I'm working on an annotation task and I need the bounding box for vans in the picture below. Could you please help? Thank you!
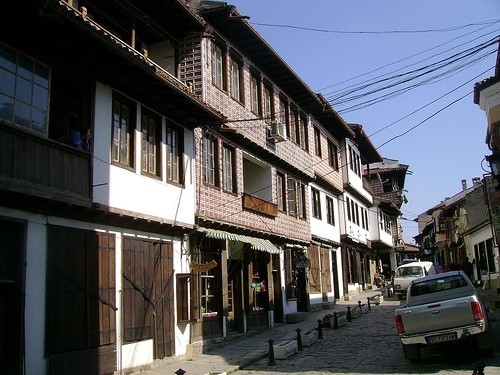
[393,261,437,301]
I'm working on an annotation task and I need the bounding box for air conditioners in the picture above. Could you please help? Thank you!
[270,120,287,142]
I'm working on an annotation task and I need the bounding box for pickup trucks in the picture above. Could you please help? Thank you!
[395,270,495,365]
